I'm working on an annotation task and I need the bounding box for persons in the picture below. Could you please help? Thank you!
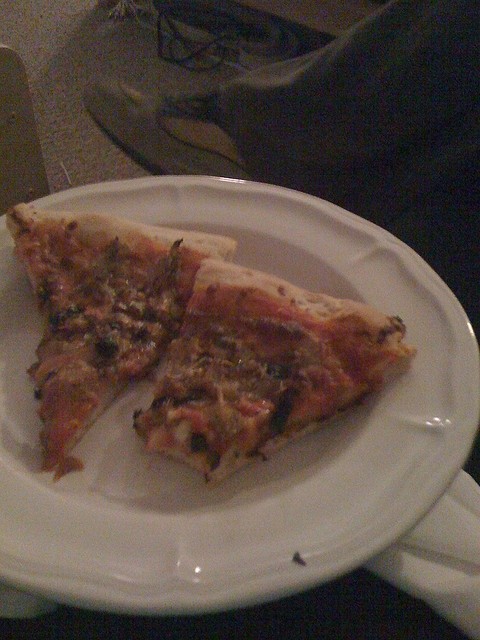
[88,1,478,343]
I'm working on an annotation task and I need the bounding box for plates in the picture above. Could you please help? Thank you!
[0,174,479,617]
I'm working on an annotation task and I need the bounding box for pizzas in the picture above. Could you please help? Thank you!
[5,208,237,473]
[134,259,419,494]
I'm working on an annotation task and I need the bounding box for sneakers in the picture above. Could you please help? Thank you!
[83,79,255,178]
